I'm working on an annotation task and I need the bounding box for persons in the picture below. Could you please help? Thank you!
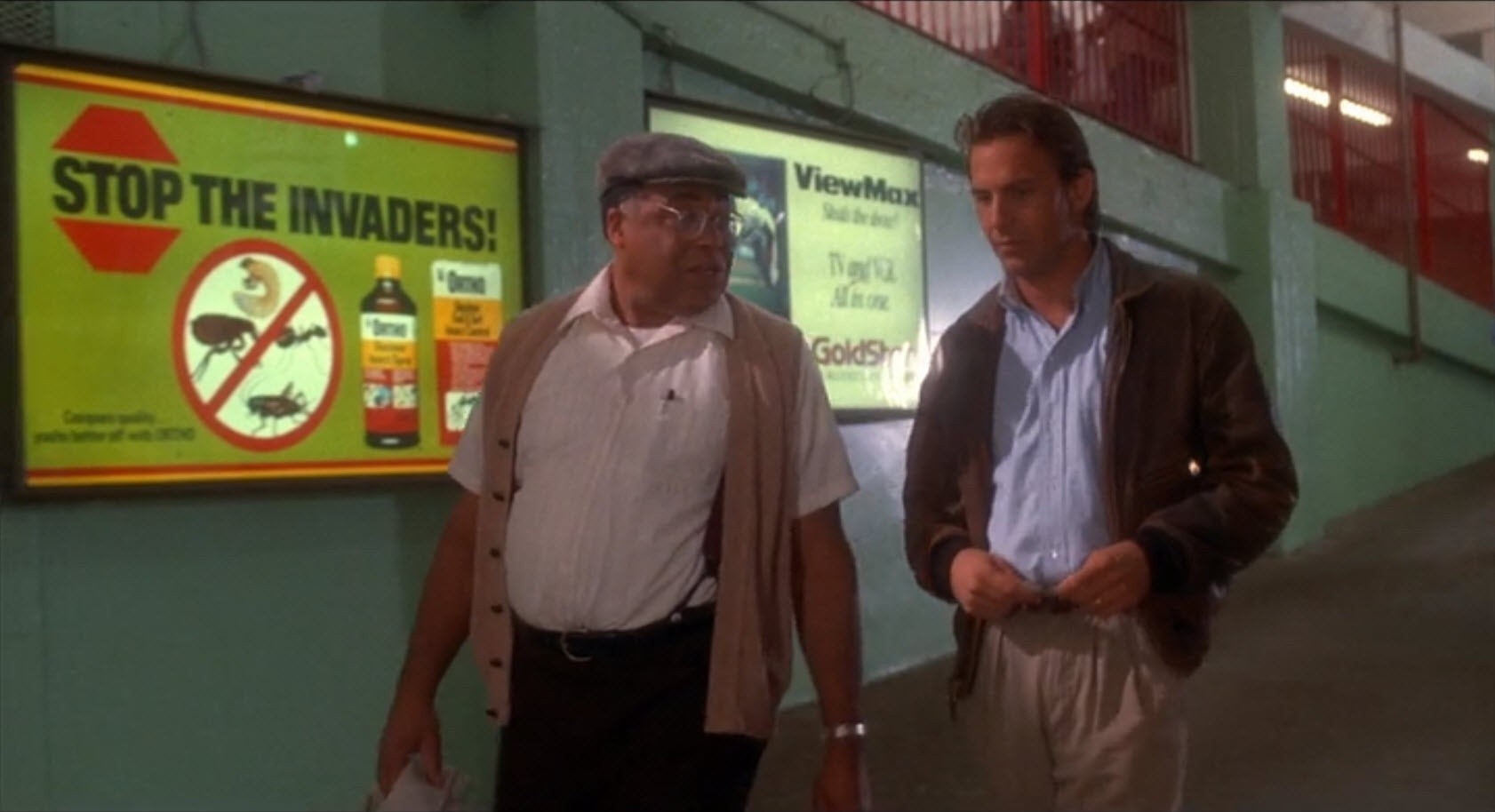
[903,92,1299,812]
[377,132,875,812]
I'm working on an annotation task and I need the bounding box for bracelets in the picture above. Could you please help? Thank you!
[821,721,867,740]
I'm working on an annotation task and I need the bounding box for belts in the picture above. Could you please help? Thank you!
[518,603,718,662]
[1011,593,1077,616]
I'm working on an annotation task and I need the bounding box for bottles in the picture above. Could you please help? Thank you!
[359,254,422,450]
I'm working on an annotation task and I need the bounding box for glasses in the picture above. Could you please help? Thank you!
[615,193,744,246]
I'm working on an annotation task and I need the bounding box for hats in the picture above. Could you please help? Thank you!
[597,134,744,201]
